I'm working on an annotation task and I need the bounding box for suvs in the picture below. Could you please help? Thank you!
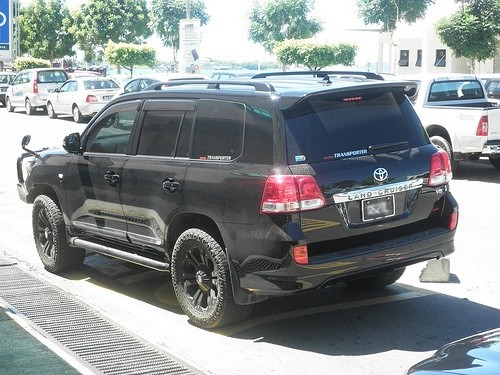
[17,71,460,330]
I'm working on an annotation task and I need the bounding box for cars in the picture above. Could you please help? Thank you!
[480,78,500,100]
[67,73,98,78]
[0,72,17,105]
[109,74,209,128]
[45,76,124,123]
[211,71,257,80]
[326,72,394,81]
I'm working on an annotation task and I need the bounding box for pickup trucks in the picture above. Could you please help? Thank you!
[384,76,500,171]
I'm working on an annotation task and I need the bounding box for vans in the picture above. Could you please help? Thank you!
[5,68,72,115]
